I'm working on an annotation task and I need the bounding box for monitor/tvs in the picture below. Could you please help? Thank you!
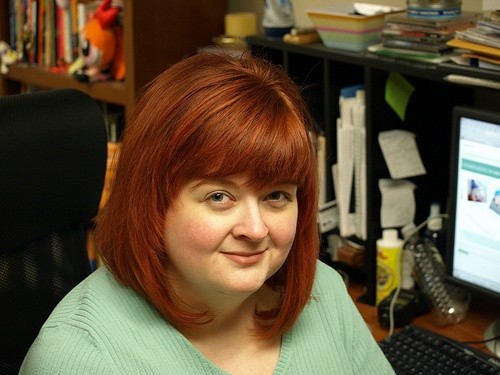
[444,107,500,297]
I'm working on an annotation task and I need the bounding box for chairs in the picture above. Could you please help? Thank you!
[0,88,108,375]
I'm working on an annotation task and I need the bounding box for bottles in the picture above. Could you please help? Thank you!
[376,229,404,308]
[401,222,467,326]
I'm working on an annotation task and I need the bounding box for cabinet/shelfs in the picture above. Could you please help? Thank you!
[0,0,228,129]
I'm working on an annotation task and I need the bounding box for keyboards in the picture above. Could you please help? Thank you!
[378,325,500,375]
